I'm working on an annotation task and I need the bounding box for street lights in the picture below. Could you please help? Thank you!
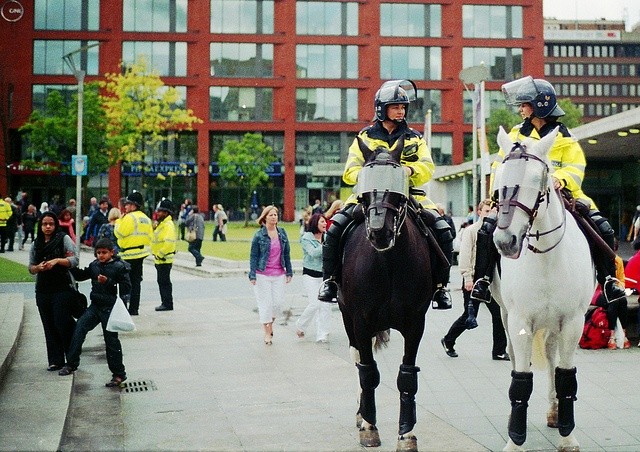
[58,37,106,270]
[460,61,490,222]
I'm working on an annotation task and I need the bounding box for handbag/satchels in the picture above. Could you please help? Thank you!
[184,214,196,242]
[57,286,87,317]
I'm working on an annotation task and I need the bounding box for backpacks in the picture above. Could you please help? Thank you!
[579,308,611,350]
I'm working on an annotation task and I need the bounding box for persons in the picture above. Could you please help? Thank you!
[180,198,194,233]
[184,205,205,267]
[247,204,294,346]
[470,75,626,303]
[22,204,36,248]
[620,209,628,241]
[439,208,456,239]
[177,204,187,241]
[97,207,121,256]
[438,198,510,363]
[218,203,228,241]
[26,212,81,371]
[592,237,631,350]
[0,196,13,252]
[58,237,132,389]
[88,196,100,217]
[460,205,479,227]
[323,199,344,232]
[19,192,29,213]
[627,205,640,241]
[152,197,177,312]
[101,194,113,210]
[294,213,329,344]
[85,199,110,247]
[316,78,454,311]
[3,197,18,251]
[298,215,304,242]
[312,199,323,216]
[66,199,76,235]
[49,195,66,214]
[624,235,640,348]
[37,201,52,220]
[212,204,224,241]
[56,209,76,246]
[14,200,24,251]
[112,191,154,317]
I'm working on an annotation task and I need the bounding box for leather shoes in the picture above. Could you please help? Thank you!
[47,363,64,371]
[318,285,336,302]
[603,283,625,301]
[432,292,451,308]
[472,283,490,301]
[155,304,173,310]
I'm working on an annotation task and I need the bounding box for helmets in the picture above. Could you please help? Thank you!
[371,78,417,122]
[502,75,566,118]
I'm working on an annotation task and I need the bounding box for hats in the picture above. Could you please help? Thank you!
[123,192,143,208]
[156,199,176,216]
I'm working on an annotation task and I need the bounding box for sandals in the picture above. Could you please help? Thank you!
[106,375,128,386]
[59,365,77,375]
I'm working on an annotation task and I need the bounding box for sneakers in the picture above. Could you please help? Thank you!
[493,353,511,358]
[264,334,272,344]
[441,338,458,357]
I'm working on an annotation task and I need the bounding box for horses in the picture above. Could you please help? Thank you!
[328,137,454,452]
[486,124,600,452]
